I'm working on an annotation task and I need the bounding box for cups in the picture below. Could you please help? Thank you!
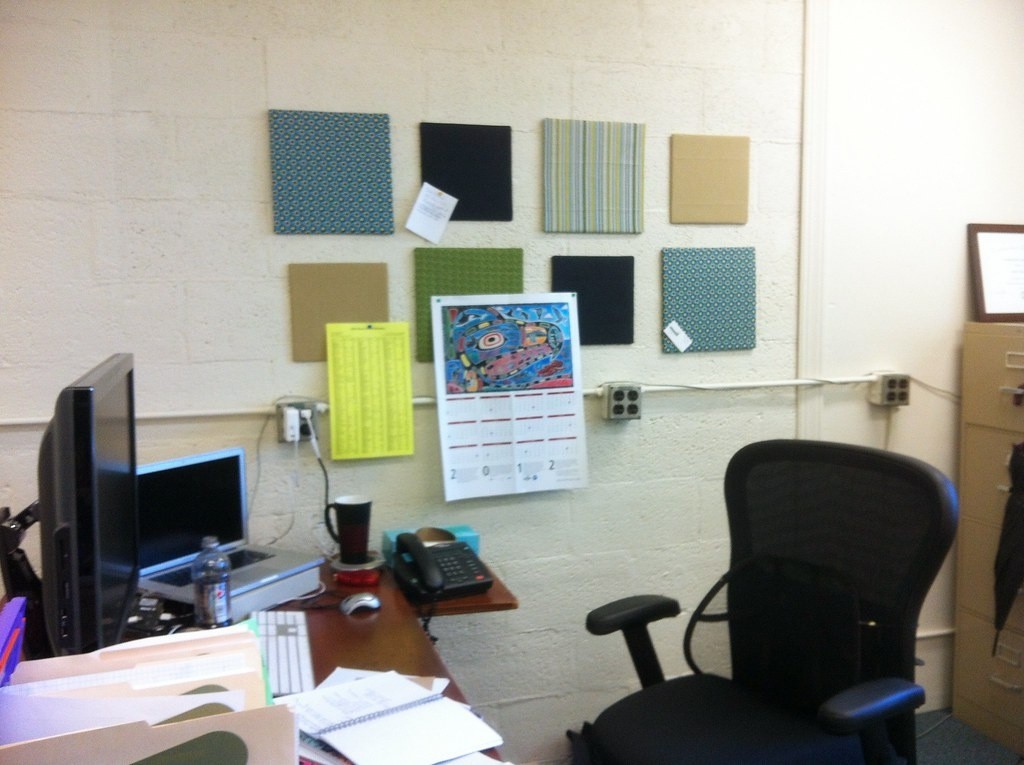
[324,496,371,565]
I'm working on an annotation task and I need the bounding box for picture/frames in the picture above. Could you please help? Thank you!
[966,222,1024,322]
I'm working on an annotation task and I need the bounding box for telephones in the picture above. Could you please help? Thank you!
[390,532,493,607]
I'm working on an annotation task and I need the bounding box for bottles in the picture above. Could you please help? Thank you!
[191,537,233,629]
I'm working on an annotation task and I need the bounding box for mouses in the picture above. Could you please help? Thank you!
[339,592,382,616]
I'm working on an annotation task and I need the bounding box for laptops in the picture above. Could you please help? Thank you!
[134,447,326,604]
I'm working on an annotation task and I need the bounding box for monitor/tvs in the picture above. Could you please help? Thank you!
[0,353,141,659]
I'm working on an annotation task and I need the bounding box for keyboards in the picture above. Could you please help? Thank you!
[249,611,315,697]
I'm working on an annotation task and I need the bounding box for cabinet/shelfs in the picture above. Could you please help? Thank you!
[948,315,1024,754]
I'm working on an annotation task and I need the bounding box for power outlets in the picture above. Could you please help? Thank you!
[276,401,318,446]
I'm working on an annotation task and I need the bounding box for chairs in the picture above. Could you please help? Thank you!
[581,439,960,764]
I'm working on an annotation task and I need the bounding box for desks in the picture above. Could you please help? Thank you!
[0,558,520,764]
